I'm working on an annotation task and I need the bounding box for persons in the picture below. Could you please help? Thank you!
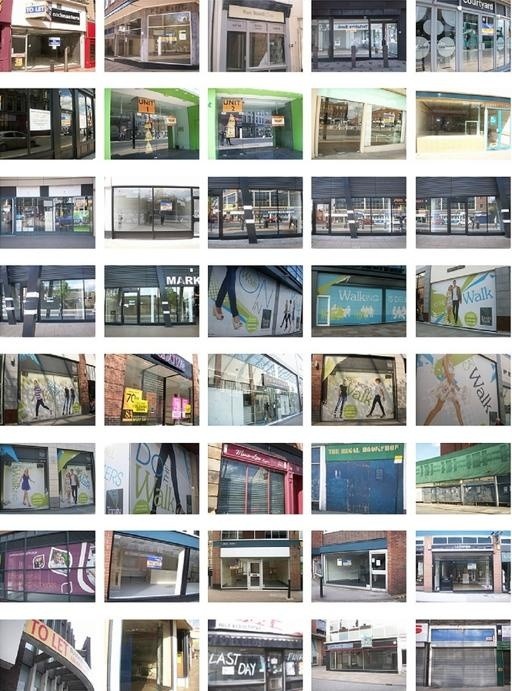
[333,376,347,421]
[211,265,242,329]
[31,380,53,421]
[292,300,295,330]
[149,444,185,514]
[365,376,389,418]
[444,284,455,323]
[284,299,292,333]
[69,388,76,416]
[63,472,72,506]
[280,299,289,331]
[423,352,466,426]
[62,387,70,416]
[223,126,234,146]
[50,551,69,576]
[452,279,462,324]
[18,467,36,508]
[70,467,80,505]
